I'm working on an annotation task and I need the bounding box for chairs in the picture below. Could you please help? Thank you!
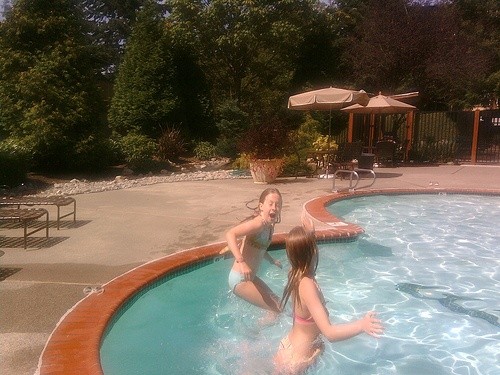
[0,195,77,230]
[291,139,360,179]
[0,208,49,250]
[376,140,398,170]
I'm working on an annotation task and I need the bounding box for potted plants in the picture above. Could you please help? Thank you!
[236,114,296,185]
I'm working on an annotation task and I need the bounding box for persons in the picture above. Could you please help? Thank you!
[225,188,283,310]
[279,226,385,361]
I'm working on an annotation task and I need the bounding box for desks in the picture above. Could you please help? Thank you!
[306,149,335,174]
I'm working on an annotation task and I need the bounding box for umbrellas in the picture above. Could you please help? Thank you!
[287,86,370,165]
[341,90,416,163]
